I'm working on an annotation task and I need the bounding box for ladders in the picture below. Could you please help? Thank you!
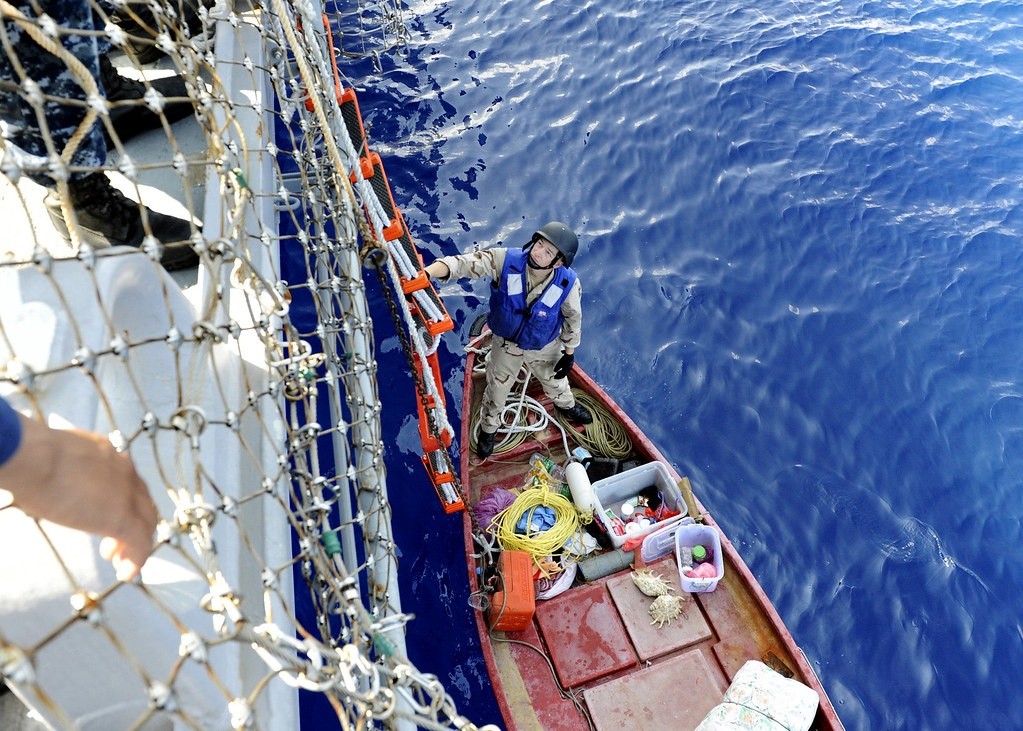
[292,0,468,515]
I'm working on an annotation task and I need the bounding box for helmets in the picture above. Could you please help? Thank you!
[531,221,579,270]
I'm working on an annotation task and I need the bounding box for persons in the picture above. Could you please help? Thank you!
[1,0,205,273]
[100,0,215,64]
[0,396,156,697]
[407,222,593,459]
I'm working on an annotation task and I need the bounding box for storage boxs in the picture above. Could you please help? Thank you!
[675,522,725,596]
[590,460,688,544]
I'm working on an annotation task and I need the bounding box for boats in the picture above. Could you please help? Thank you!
[460,313,848,731]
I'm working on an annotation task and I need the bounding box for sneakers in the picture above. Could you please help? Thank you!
[554,402,592,424]
[477,423,505,458]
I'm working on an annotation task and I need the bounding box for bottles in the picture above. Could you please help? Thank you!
[694,545,707,562]
[527,477,571,497]
[621,502,651,534]
[529,453,569,484]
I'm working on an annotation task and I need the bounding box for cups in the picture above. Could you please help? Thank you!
[681,545,694,567]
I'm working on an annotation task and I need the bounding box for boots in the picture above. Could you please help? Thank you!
[43,169,204,271]
[94,53,204,153]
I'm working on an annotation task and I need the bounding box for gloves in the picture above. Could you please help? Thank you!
[404,270,430,304]
[553,349,575,379]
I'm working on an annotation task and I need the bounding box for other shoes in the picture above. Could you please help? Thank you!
[137,15,203,66]
